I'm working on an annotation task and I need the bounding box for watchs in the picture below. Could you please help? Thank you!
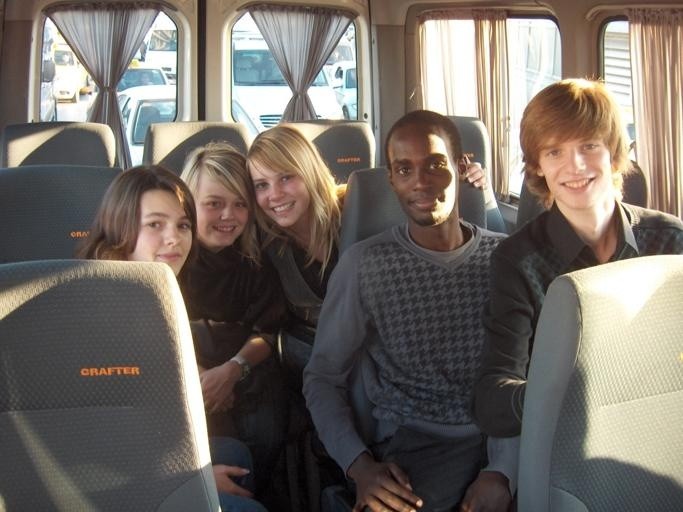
[230,353,251,382]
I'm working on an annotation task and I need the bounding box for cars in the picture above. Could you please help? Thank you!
[116,85,265,171]
[231,25,358,129]
[41,17,178,123]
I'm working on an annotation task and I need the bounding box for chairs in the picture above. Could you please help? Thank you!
[517,252,683,511]
[339,160,648,263]
[0,165,223,512]
[1,117,493,180]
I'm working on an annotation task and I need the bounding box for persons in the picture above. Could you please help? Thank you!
[179,140,292,511]
[245,126,485,511]
[298,109,518,512]
[73,166,262,511]
[469,80,681,434]
